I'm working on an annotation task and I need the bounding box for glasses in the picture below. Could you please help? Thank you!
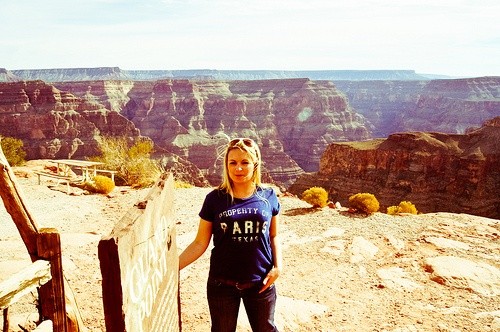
[227,137,256,148]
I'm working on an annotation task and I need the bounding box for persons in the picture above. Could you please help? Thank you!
[179,136,282,332]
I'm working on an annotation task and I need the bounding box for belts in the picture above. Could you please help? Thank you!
[207,276,259,291]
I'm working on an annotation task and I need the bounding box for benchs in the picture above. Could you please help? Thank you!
[33,169,75,195]
[74,167,118,185]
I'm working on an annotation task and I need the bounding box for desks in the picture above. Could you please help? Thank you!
[48,158,106,184]
